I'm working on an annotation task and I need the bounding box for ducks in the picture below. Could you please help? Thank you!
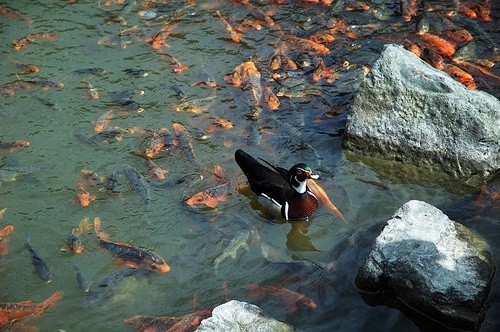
[233,149,324,223]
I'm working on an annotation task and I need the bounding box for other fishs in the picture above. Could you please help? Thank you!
[1,0,499,332]
